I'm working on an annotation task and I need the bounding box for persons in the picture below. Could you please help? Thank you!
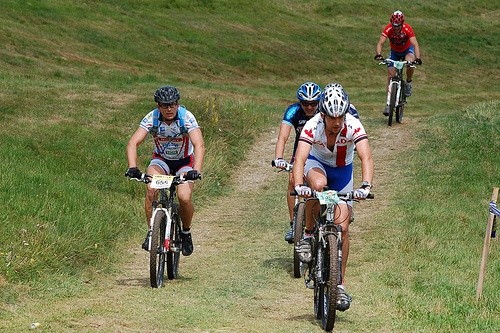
[293,82,374,312]
[374,10,422,115]
[275,82,322,241]
[125,86,206,256]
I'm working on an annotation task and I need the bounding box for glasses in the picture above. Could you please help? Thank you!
[392,24,402,27]
[300,99,316,108]
[159,101,177,109]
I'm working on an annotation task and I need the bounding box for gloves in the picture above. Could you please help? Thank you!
[186,170,198,180]
[127,167,141,179]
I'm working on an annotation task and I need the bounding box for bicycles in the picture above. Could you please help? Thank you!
[271,161,374,333]
[378,58,421,125]
[124,169,201,288]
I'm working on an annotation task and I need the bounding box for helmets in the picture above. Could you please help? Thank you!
[319,88,350,116]
[323,82,344,91]
[390,10,405,24]
[154,86,179,104]
[297,81,322,102]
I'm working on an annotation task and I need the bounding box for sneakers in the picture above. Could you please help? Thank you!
[404,81,413,96]
[142,231,151,248]
[181,231,193,256]
[335,286,352,310]
[285,225,293,240]
[295,234,312,262]
[383,105,390,115]
